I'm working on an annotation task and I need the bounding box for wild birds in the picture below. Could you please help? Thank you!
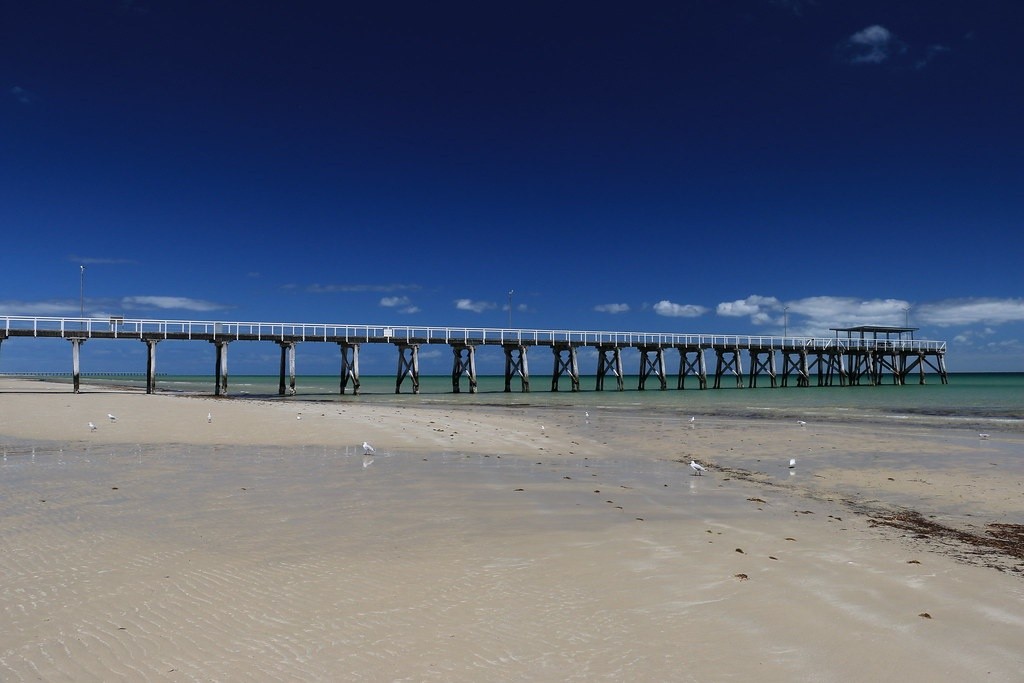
[108,414,116,421]
[207,412,212,423]
[690,461,709,475]
[361,441,375,454]
[979,434,991,440]
[296,416,302,420]
[585,412,589,418]
[797,421,807,428]
[689,417,694,423]
[790,459,796,468]
[541,426,544,430]
[88,422,97,432]
